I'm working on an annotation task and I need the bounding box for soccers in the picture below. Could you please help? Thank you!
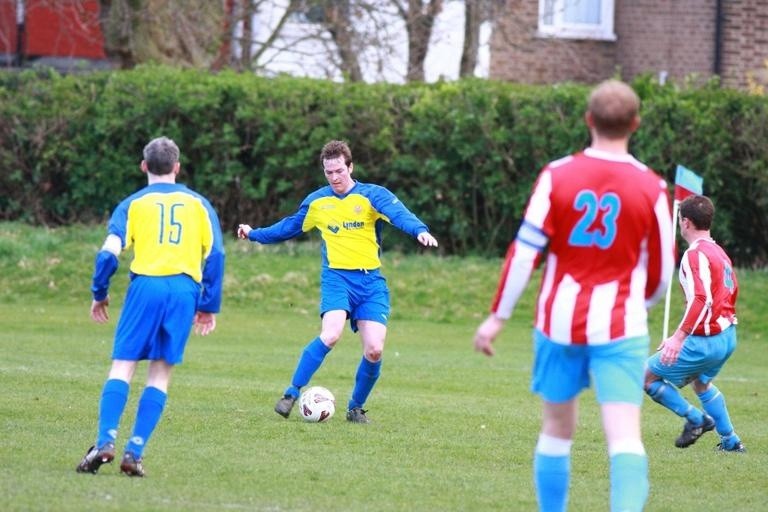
[299,386,335,422]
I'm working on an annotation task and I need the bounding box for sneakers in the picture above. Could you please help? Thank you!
[713,440,747,455]
[76,441,116,474]
[346,405,371,423]
[673,411,715,448]
[119,451,146,477]
[274,394,295,418]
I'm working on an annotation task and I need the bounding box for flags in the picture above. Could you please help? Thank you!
[675,164,704,203]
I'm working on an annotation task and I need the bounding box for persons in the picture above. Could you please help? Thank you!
[642,193,747,453]
[470,77,676,512]
[77,135,227,477]
[235,139,440,426]
